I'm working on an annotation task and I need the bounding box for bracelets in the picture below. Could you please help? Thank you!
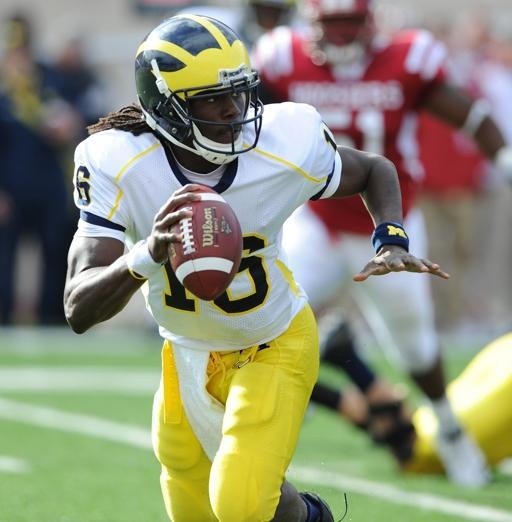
[493,144,512,182]
[370,221,411,253]
[126,239,163,279]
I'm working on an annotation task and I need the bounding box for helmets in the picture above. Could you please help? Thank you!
[316,0,368,47]
[133,12,265,166]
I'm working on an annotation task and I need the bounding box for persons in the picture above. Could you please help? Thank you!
[61,9,453,520]
[239,1,306,53]
[313,307,418,454]
[245,1,512,493]
[41,37,110,137]
[1,12,80,333]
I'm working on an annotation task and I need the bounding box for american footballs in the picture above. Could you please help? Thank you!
[168,184,244,301]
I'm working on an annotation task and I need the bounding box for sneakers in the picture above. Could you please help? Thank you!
[356,389,493,496]
